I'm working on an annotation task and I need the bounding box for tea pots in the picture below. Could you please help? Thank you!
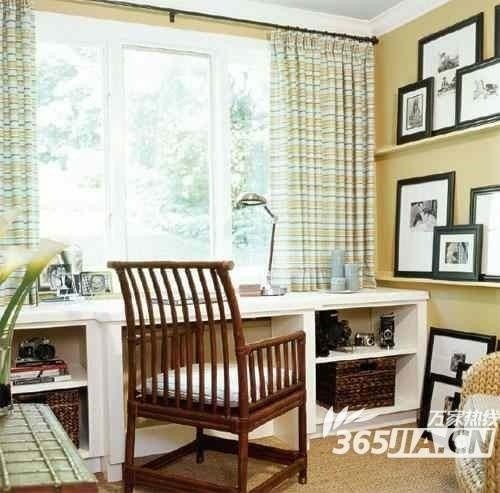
[49,262,76,299]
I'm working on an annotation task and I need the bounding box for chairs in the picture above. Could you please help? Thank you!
[107,256,309,490]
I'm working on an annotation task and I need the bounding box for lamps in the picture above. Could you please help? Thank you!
[236,193,288,295]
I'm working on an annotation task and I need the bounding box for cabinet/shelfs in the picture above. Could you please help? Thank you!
[371,115,500,289]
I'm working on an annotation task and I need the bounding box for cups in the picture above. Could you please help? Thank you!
[280,287,289,294]
[270,279,280,295]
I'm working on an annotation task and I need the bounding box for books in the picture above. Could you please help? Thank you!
[10,359,72,385]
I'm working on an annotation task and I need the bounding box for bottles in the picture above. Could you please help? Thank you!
[331,249,363,292]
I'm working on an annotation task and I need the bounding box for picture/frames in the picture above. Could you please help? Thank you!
[433,224,483,282]
[470,184,500,282]
[454,57,500,131]
[416,327,499,445]
[396,77,434,147]
[393,171,456,277]
[418,11,484,140]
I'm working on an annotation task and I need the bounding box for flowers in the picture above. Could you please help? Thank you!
[0,240,64,405]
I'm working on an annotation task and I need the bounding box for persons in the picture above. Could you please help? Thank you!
[89,274,106,292]
[411,201,437,226]
[50,266,71,289]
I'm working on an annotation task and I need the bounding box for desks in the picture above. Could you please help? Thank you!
[1,286,430,483]
[0,404,99,493]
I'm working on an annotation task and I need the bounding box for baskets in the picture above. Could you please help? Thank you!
[319,358,396,413]
[14,390,81,449]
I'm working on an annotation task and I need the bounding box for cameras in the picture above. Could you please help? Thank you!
[355,332,375,346]
[378,315,394,346]
[17,335,57,363]
